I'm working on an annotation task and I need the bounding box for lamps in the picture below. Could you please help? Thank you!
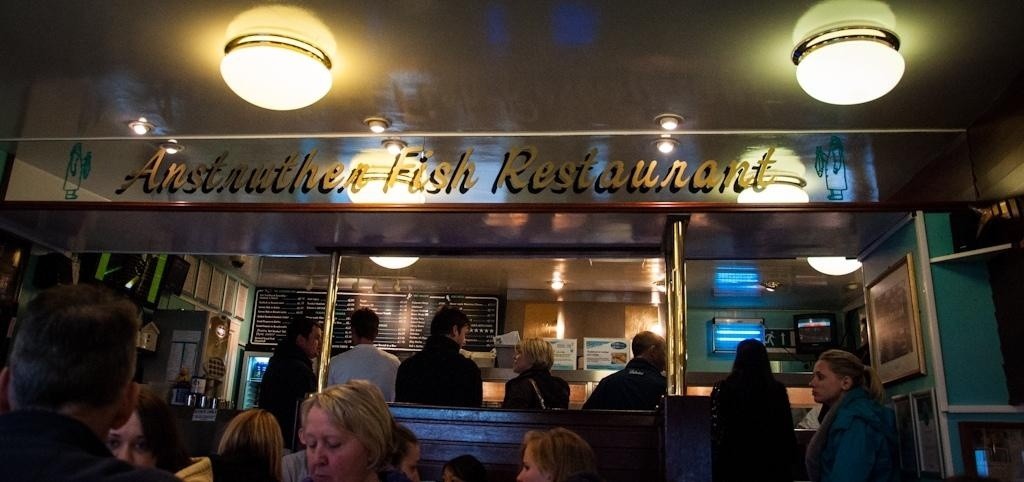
[369,256,420,270]
[807,257,862,276]
[790,24,904,105]
[219,33,333,111]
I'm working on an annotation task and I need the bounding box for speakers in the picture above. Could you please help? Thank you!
[161,254,191,298]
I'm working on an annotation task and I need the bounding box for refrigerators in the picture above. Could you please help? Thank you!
[236,351,277,410]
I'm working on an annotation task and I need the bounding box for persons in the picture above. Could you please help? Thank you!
[1,283,186,481]
[804,350,903,482]
[258,318,323,481]
[105,384,212,482]
[711,339,799,482]
[495,337,570,410]
[395,307,483,409]
[581,330,666,410]
[214,379,600,481]
[326,307,401,402]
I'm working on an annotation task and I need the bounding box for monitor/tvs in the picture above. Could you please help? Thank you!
[792,312,840,355]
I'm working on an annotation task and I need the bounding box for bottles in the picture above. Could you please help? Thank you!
[254,360,267,378]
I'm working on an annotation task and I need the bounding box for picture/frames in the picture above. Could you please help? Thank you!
[891,391,921,481]
[912,387,946,482]
[863,252,928,387]
